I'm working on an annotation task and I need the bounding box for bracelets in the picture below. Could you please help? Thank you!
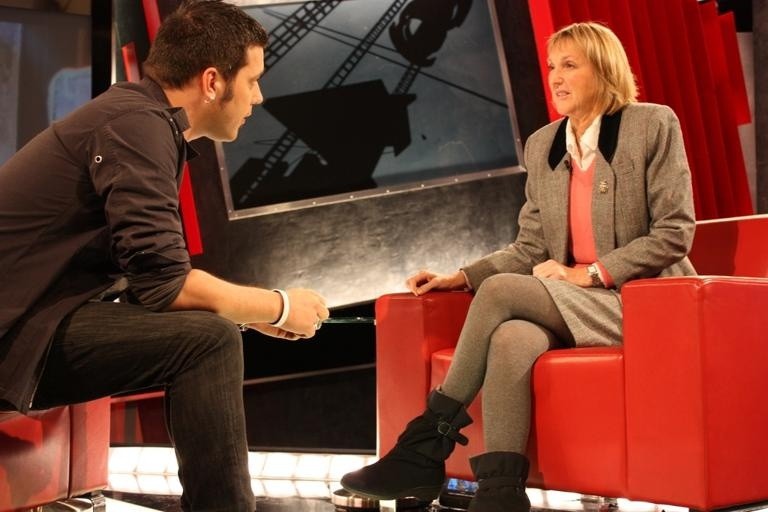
[271,288,289,326]
[240,322,248,331]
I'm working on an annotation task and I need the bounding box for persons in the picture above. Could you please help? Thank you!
[339,23,697,511]
[1,0,331,512]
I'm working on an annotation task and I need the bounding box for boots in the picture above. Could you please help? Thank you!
[339,383,475,504]
[468,449,532,512]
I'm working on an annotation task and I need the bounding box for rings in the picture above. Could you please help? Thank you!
[315,318,323,330]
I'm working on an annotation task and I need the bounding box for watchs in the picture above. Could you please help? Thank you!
[586,265,602,287]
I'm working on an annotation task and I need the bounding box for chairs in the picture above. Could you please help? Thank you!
[367,211,767,510]
[0,393,112,511]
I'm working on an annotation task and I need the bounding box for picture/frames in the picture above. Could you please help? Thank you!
[175,2,530,223]
